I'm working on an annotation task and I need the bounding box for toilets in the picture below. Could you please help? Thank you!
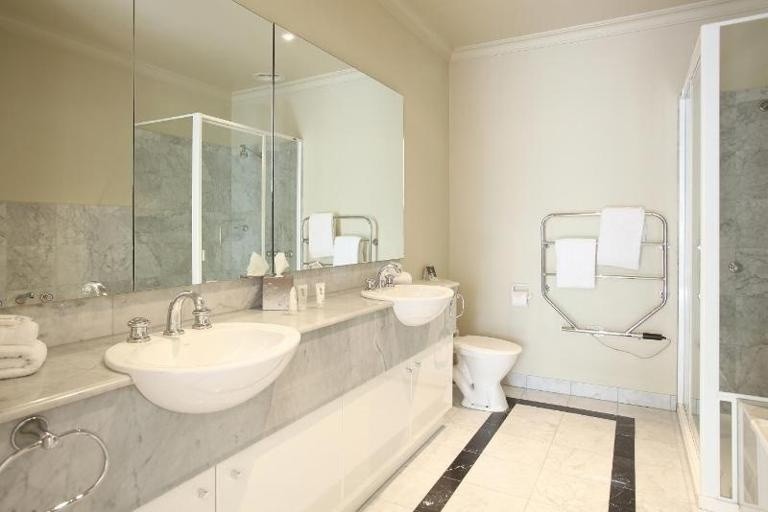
[412,279,523,413]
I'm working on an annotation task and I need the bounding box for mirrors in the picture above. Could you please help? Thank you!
[0,1,134,303]
[272,18,410,280]
[133,0,271,299]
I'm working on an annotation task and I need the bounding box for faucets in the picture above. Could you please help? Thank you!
[80,280,111,298]
[374,262,402,288]
[162,290,212,337]
[308,260,324,268]
[14,292,35,305]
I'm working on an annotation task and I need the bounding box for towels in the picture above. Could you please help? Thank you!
[304,210,339,259]
[330,234,367,266]
[1,313,42,345]
[549,233,600,291]
[1,340,49,381]
[595,204,647,274]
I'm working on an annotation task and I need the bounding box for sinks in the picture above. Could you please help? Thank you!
[103,322,302,415]
[361,284,455,327]
